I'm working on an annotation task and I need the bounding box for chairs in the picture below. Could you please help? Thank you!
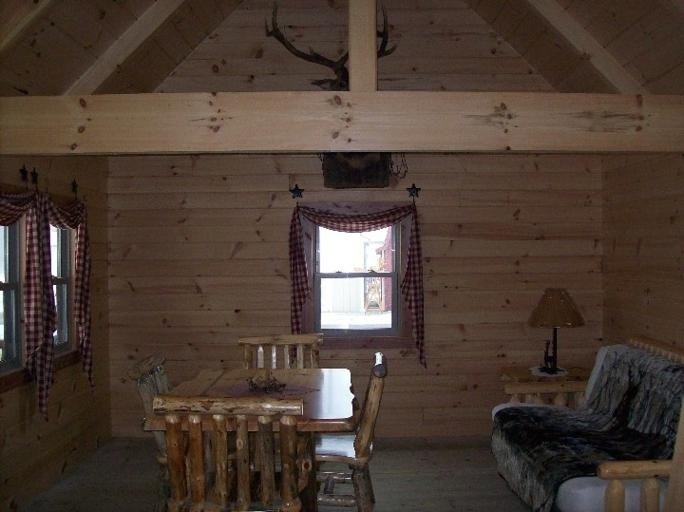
[133,349,208,495]
[239,333,326,374]
[300,352,387,509]
[149,388,303,511]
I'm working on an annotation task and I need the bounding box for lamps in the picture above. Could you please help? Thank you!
[528,288,585,375]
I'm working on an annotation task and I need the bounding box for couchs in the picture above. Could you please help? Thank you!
[491,336,684,512]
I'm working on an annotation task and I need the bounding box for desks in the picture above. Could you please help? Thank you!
[498,364,588,409]
[146,367,354,499]
